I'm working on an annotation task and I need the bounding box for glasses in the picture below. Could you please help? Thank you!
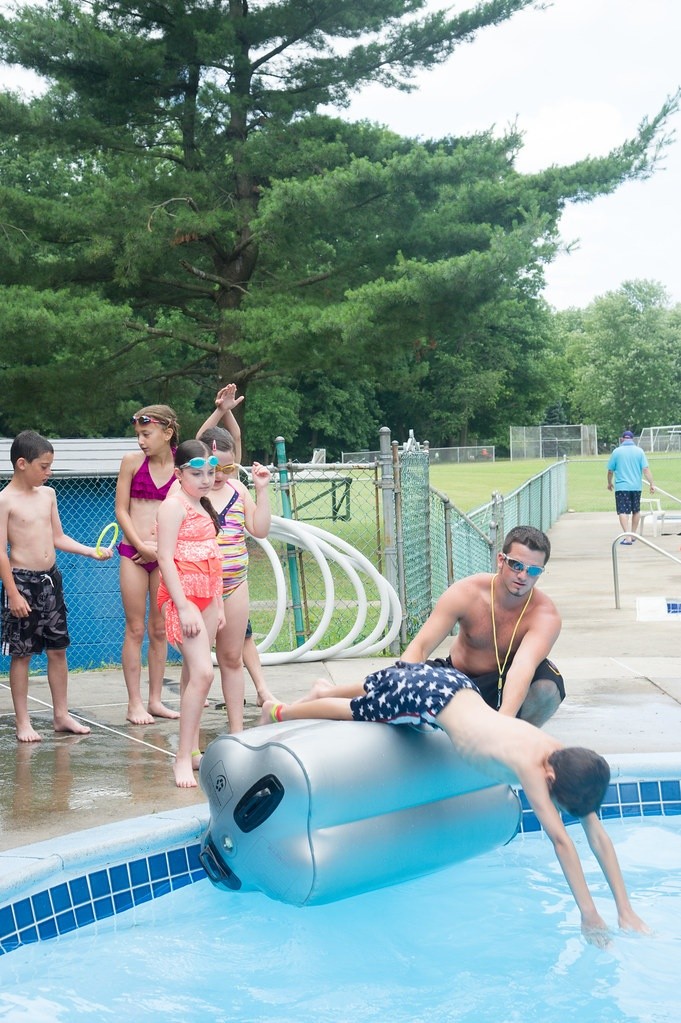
[215,463,236,474]
[503,552,546,577]
[130,416,165,424]
[179,455,219,469]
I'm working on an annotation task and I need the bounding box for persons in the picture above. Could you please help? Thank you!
[258,664,649,936]
[0,430,114,741]
[195,383,281,705]
[157,440,226,787]
[116,405,180,725]
[607,430,654,545]
[398,525,565,728]
[169,428,271,733]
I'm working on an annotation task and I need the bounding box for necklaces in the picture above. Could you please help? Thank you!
[491,573,533,709]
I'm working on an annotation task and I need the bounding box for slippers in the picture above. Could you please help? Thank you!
[620,538,632,545]
[630,538,636,542]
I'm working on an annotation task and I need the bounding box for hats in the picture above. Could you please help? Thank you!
[622,430,634,439]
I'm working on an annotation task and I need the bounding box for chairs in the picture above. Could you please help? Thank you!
[639,498,665,537]
